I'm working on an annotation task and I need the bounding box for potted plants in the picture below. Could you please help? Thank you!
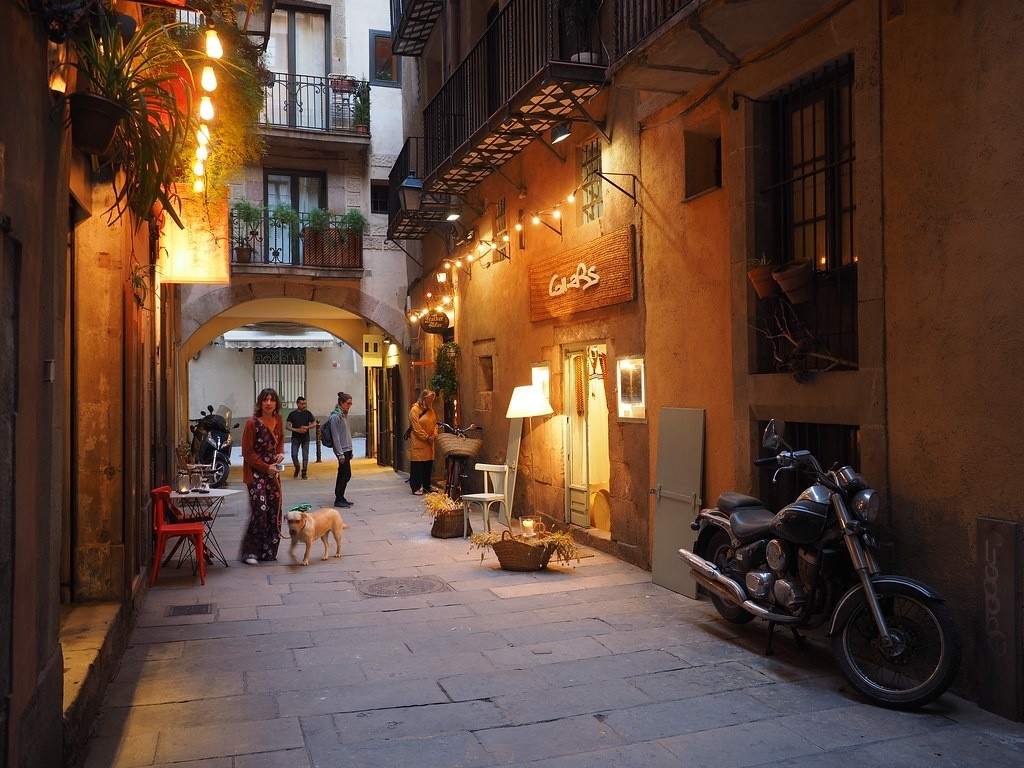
[44,0,272,312]
[350,97,371,135]
[428,341,462,402]
[331,74,357,93]
[735,250,779,299]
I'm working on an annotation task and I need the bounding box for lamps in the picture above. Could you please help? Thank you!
[383,337,391,344]
[550,121,571,144]
[506,384,554,515]
[396,170,422,212]
[446,209,461,220]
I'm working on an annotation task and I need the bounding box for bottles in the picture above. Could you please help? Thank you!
[175,470,211,492]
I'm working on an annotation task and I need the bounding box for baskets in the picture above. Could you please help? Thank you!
[435,432,485,459]
[492,530,557,571]
[431,508,474,537]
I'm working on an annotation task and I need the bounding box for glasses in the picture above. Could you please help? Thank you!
[344,402,352,406]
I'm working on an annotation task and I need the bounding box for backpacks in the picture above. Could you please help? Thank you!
[319,412,342,448]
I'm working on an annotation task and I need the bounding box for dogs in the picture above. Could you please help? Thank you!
[283,507,349,566]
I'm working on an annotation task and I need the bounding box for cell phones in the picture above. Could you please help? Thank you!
[276,465,285,472]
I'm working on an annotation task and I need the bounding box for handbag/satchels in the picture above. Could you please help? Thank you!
[404,427,413,440]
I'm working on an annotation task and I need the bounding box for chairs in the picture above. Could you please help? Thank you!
[461,463,513,540]
[177,447,213,506]
[149,486,212,591]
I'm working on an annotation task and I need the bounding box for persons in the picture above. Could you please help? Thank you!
[242,388,285,565]
[329,392,354,507]
[286,397,317,479]
[408,390,438,495]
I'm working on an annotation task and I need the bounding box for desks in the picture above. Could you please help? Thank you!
[169,488,245,577]
[177,464,211,475]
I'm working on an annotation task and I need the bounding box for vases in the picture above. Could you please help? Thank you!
[235,247,251,262]
[773,256,817,304]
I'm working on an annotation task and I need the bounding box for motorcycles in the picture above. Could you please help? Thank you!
[190,405,240,488]
[677,417,961,712]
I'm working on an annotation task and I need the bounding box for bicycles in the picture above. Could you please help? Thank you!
[438,422,483,500]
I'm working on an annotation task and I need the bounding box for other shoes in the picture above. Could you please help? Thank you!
[430,488,439,493]
[413,490,423,495]
[348,501,354,506]
[245,558,258,565]
[334,499,351,508]
[302,472,307,480]
[294,468,299,478]
